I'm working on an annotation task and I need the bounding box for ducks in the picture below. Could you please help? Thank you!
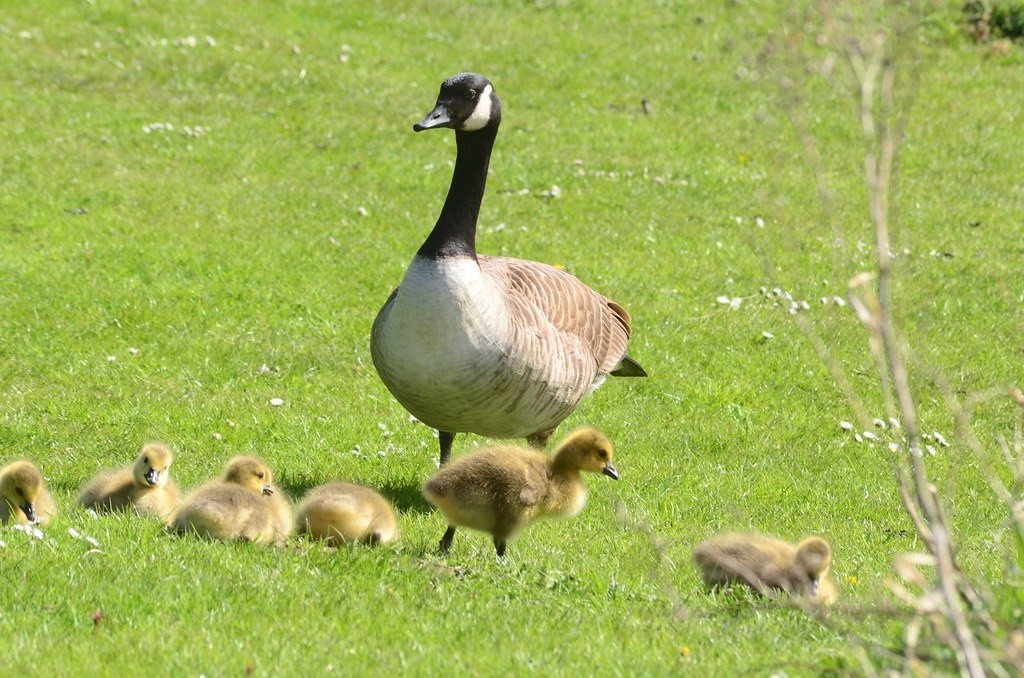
[0,427,830,603]
[369,73,648,559]
